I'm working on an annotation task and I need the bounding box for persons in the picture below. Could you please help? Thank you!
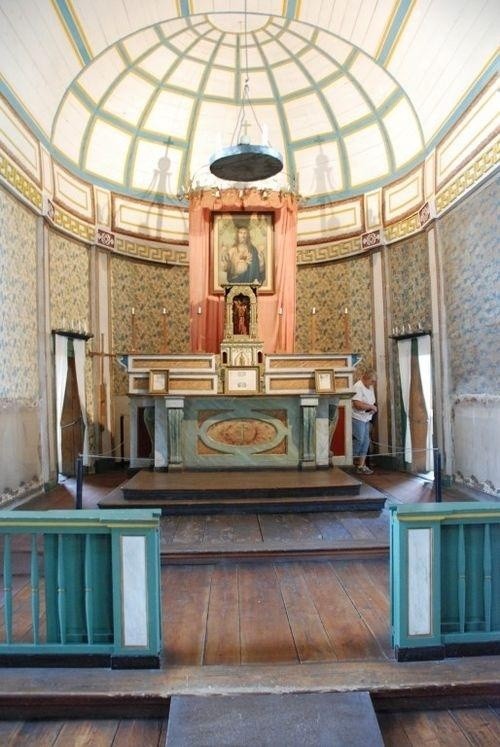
[349,367,378,476]
[223,224,263,286]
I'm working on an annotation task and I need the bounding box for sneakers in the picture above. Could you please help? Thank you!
[353,464,376,476]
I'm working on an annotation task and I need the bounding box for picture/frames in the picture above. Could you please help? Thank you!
[223,365,262,396]
[315,369,336,394]
[149,369,169,396]
[209,211,276,295]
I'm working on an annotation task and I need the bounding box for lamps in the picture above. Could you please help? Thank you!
[209,0,284,182]
